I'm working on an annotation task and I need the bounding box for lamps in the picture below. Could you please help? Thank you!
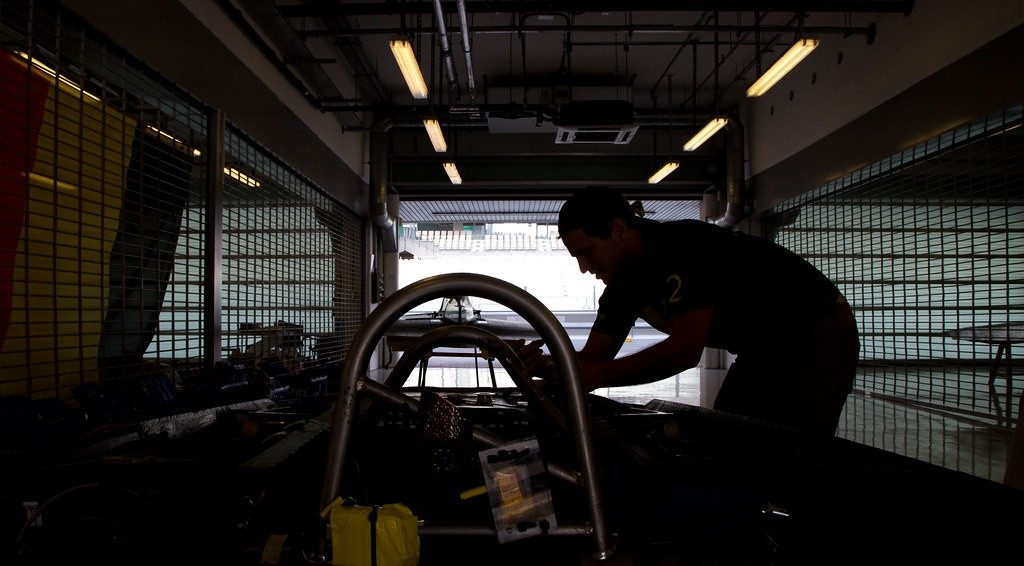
[680,112,728,152]
[422,104,447,152]
[389,33,429,99]
[741,18,820,98]
[647,157,679,184]
[442,156,462,184]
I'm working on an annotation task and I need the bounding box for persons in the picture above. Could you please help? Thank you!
[502,186,860,565]
